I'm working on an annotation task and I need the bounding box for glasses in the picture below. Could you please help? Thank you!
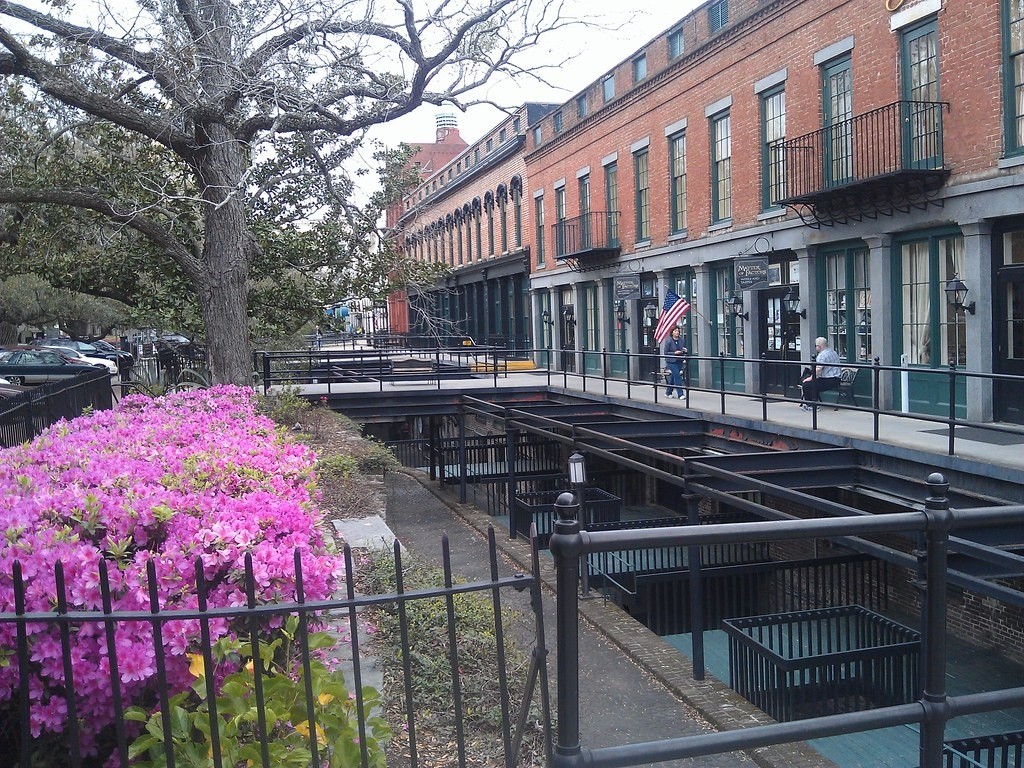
[815,344,819,348]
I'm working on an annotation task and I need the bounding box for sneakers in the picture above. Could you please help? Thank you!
[675,394,686,400]
[663,393,674,399]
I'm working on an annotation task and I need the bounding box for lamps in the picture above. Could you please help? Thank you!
[783,287,806,319]
[563,306,576,326]
[644,301,659,327]
[944,272,975,315]
[614,304,630,325]
[568,453,595,600]
[728,294,749,321]
[541,310,554,326]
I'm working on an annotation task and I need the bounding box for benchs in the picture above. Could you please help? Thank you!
[649,358,688,388]
[797,365,861,411]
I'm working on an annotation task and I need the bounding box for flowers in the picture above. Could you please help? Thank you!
[0,383,350,768]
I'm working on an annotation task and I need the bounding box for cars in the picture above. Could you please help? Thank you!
[0,329,202,423]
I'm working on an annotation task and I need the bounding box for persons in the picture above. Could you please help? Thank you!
[316,325,323,348]
[664,327,688,400]
[347,321,364,339]
[801,337,843,413]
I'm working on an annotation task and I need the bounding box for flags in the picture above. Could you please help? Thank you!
[654,288,692,343]
